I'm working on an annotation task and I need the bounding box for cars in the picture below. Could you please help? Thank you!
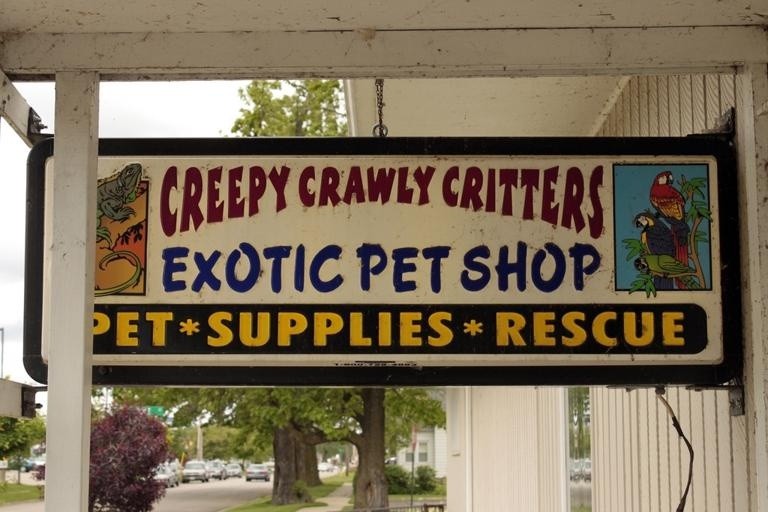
[245,462,271,482]
[0,453,36,472]
[318,462,338,472]
[146,461,242,488]
[571,456,592,483]
